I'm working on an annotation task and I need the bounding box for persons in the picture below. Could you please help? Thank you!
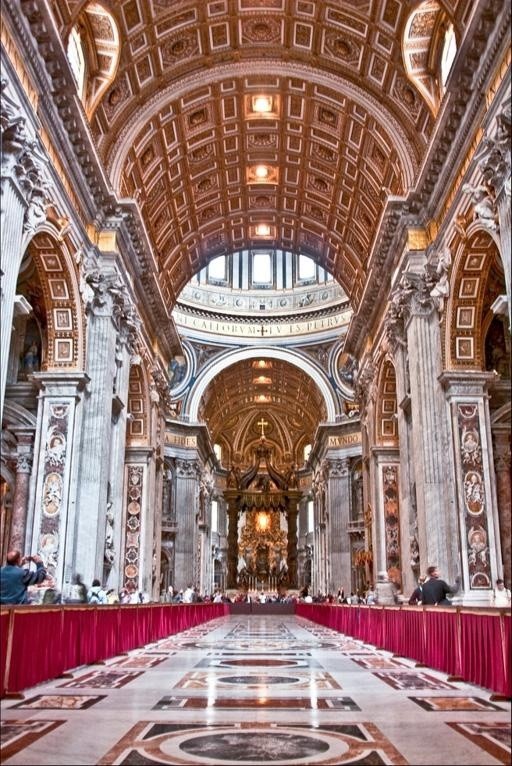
[489,579,512,608]
[23,344,39,380]
[58,343,69,359]
